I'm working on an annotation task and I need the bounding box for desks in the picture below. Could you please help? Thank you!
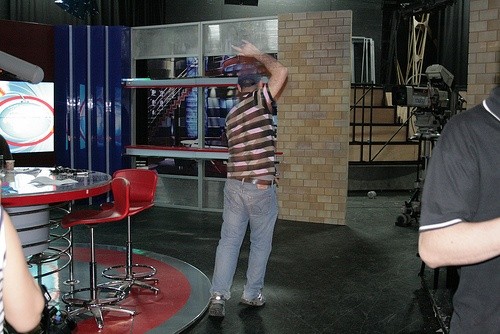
[0,166,112,334]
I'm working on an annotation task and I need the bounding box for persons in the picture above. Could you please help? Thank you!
[418,86,500,334]
[208,39,288,316]
[0,204,45,334]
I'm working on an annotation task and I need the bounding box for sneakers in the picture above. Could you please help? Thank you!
[209,294,226,316]
[240,292,266,306]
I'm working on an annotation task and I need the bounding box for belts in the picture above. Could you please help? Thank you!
[230,176,275,185]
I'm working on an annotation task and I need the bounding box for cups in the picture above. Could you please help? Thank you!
[5,160,15,170]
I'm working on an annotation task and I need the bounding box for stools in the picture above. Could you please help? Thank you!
[97,168,161,300]
[60,176,136,331]
